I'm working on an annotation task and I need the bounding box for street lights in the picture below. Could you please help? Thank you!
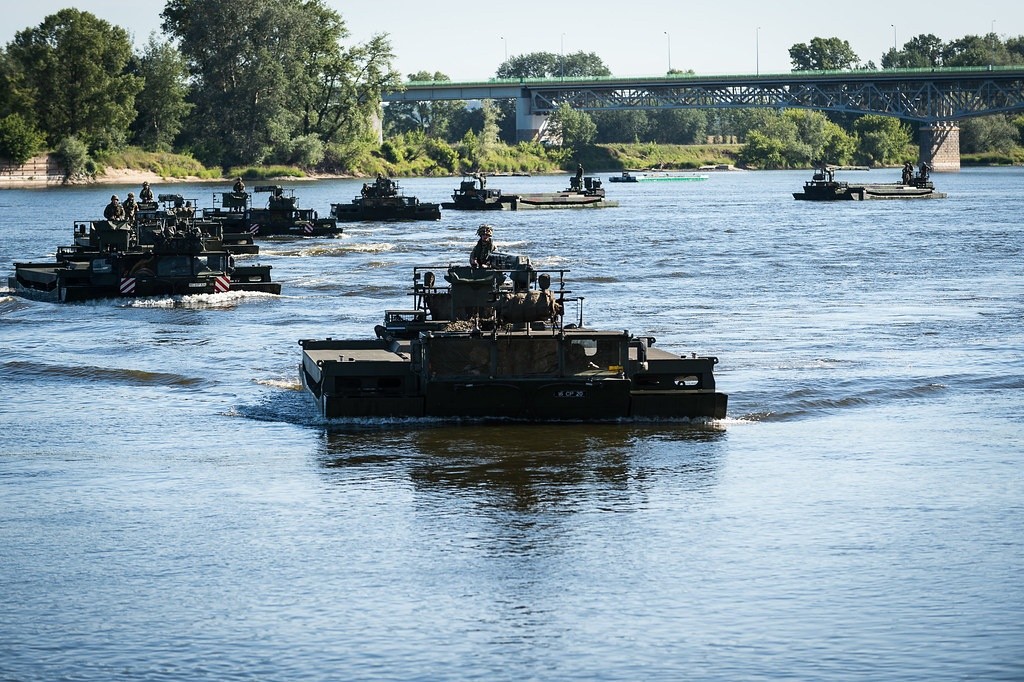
[500,38,508,78]
[757,27,761,76]
[664,32,670,77]
[892,25,896,49]
[562,32,566,78]
[992,20,996,33]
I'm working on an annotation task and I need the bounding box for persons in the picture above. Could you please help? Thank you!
[376,172,383,182]
[104,182,153,242]
[233,176,245,212]
[362,183,369,195]
[576,164,584,190]
[471,225,498,268]
[903,161,931,185]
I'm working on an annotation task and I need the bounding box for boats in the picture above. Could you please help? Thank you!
[442,173,622,208]
[330,179,442,220]
[8,192,343,301]
[609,171,638,183]
[793,161,946,202]
[298,251,728,424]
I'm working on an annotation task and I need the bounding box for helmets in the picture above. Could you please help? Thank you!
[128,192,134,197]
[111,195,118,201]
[143,181,149,186]
[905,163,909,166]
[363,183,367,186]
[378,172,382,175]
[236,177,242,181]
[577,164,582,167]
[477,224,493,237]
[923,162,926,165]
[909,161,912,164]
